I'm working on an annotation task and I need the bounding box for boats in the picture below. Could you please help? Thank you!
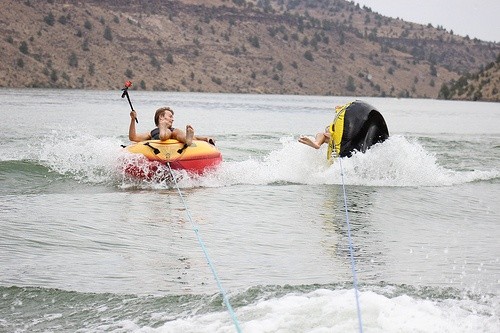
[120,137,224,182]
[327,99,390,167]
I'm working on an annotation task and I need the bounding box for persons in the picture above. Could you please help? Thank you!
[129,108,216,146]
[298,105,342,150]
